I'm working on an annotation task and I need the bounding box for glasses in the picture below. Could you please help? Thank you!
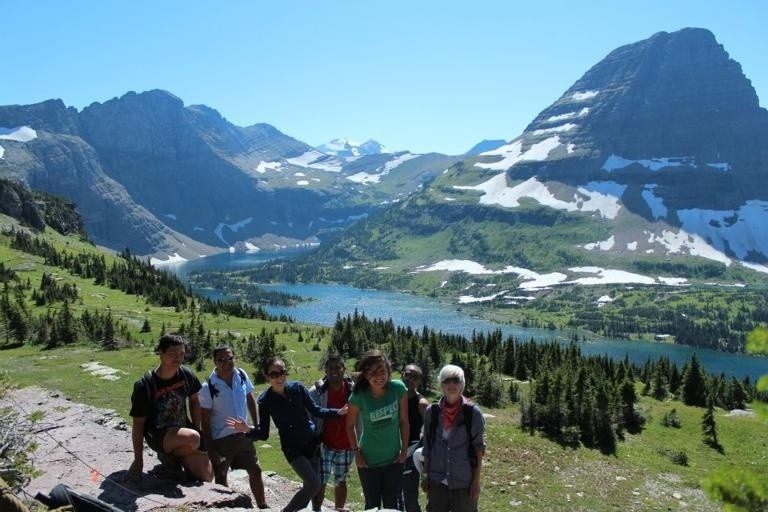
[443,378,460,384]
[217,355,234,363]
[268,369,288,380]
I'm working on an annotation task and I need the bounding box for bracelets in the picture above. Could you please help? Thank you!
[353,448,360,453]
[420,473,430,479]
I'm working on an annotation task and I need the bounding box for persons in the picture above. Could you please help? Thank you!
[420,364,487,511]
[223,355,349,512]
[345,349,411,509]
[121,334,214,484]
[306,357,355,512]
[199,344,270,509]
[396,363,431,511]
